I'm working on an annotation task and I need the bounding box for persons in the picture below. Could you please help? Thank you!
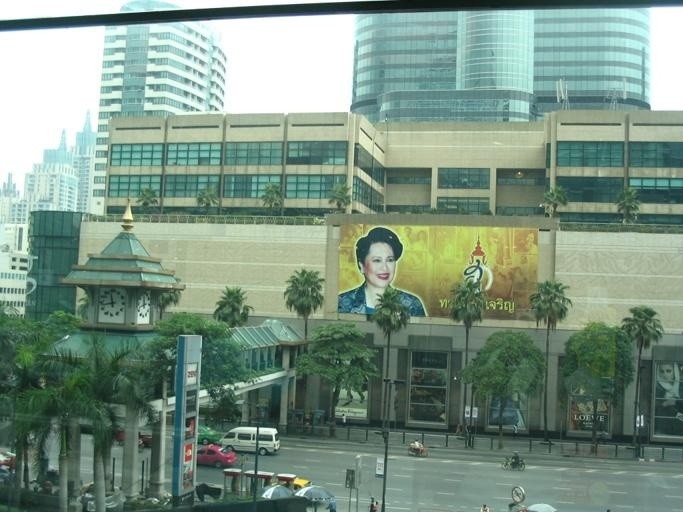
[514,424,519,439]
[325,498,338,511]
[413,439,422,453]
[480,504,490,512]
[337,227,427,317]
[340,414,348,425]
[512,452,520,469]
[656,362,683,419]
[370,497,380,511]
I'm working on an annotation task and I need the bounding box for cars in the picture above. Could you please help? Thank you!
[196,443,236,468]
[172,425,219,445]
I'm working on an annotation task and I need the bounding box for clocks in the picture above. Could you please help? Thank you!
[136,290,152,324]
[98,286,127,325]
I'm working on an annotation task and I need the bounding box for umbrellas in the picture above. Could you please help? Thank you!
[293,485,336,511]
[257,484,294,502]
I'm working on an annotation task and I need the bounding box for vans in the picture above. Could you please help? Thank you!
[217,426,279,455]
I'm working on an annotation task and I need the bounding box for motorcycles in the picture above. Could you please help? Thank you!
[501,456,525,471]
[407,443,428,457]
